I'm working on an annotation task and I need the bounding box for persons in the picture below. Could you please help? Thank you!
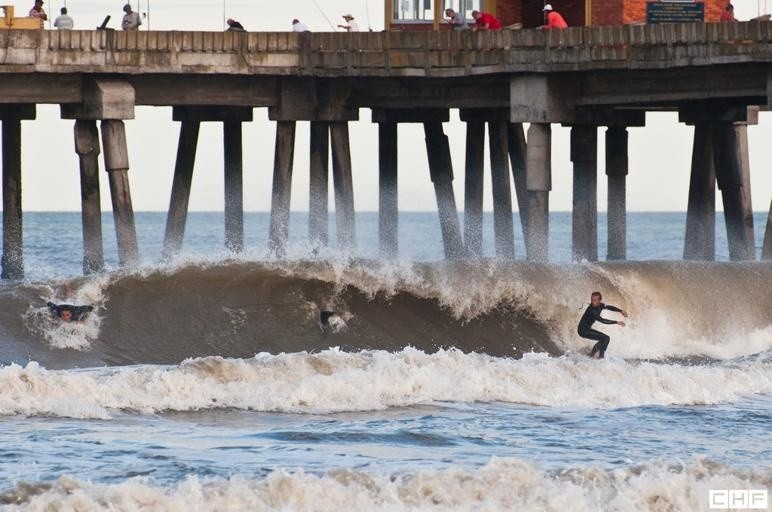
[227,19,244,30]
[121,5,142,31]
[720,4,736,22]
[54,7,75,30]
[536,4,568,31]
[578,292,629,359]
[29,1,48,30]
[337,14,361,33]
[292,19,309,32]
[472,11,502,33]
[446,8,470,30]
[46,301,94,324]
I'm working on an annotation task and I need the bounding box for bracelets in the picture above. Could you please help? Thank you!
[477,26,479,31]
[453,20,455,24]
[541,26,543,30]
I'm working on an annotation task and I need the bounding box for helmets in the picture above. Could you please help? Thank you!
[541,3,553,11]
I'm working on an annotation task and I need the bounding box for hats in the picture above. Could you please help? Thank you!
[342,13,355,20]
